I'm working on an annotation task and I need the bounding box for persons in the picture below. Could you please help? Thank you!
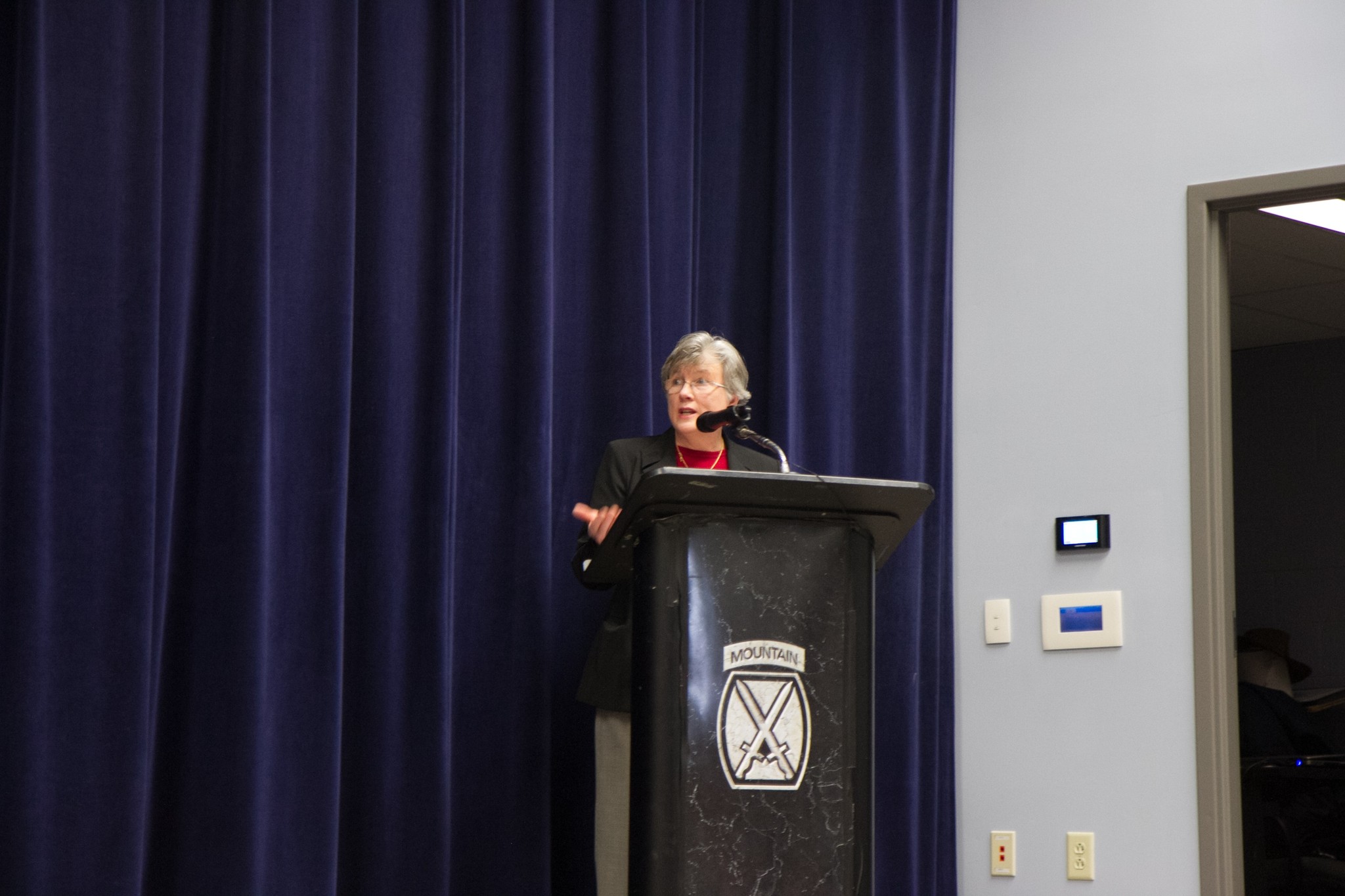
[571,329,781,896]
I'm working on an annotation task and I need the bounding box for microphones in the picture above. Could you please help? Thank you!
[696,405,753,432]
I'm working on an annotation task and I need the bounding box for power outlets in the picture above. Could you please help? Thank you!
[1066,832,1096,880]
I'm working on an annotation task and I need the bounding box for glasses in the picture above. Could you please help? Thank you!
[661,378,727,394]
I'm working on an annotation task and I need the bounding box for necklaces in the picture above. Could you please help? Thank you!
[675,436,724,469]
